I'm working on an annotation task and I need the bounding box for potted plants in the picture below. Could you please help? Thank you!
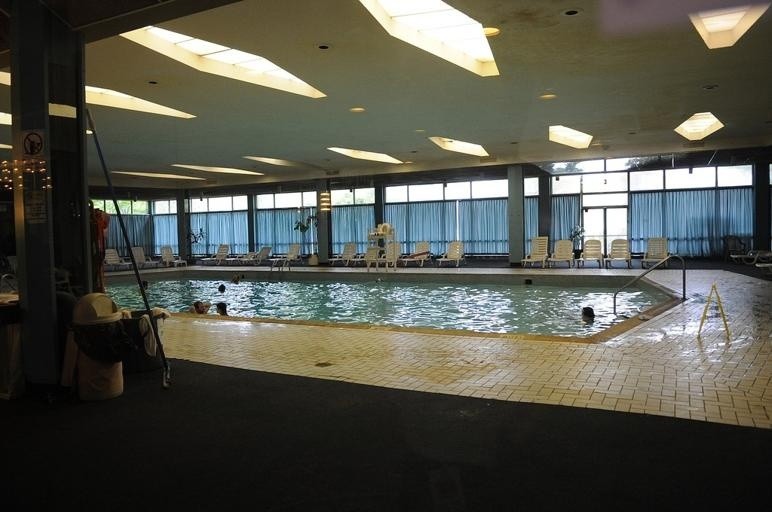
[568,225,586,259]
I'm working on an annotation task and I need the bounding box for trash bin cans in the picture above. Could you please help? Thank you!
[71,293,124,401]
[125,310,162,372]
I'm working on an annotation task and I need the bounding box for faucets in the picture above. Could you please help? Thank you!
[1,273,17,281]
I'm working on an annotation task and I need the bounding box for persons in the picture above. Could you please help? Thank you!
[189,301,211,314]
[581,307,595,322]
[217,285,226,293]
[216,303,229,316]
[142,280,148,291]
[230,274,240,284]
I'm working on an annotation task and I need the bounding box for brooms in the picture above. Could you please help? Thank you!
[83,105,172,391]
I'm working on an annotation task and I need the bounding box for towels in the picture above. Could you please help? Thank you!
[153,307,171,318]
[139,314,156,356]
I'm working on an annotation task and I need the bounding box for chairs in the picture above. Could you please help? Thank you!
[755,263,772,275]
[724,235,745,264]
[161,247,187,268]
[201,242,465,272]
[730,250,772,266]
[104,249,132,271]
[521,236,667,268]
[131,247,159,270]
